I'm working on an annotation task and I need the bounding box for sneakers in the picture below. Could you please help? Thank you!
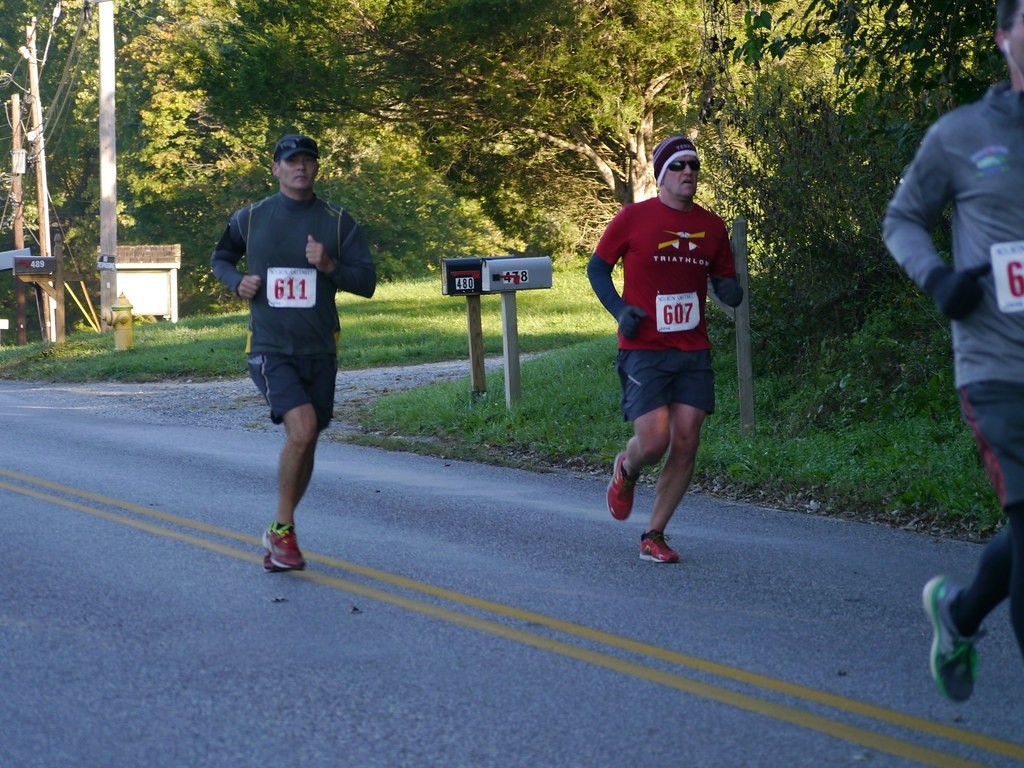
[923,575,986,702]
[606,452,641,521]
[263,521,305,572]
[639,529,679,562]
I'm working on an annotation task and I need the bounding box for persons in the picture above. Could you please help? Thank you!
[212,135,376,573]
[587,137,743,563]
[882,1,1024,703]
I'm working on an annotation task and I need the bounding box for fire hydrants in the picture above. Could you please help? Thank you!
[102,291,133,352]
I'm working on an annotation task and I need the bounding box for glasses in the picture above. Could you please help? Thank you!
[274,138,318,162]
[668,160,700,171]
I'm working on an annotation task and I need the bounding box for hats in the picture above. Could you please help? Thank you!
[274,134,321,160]
[653,137,699,187]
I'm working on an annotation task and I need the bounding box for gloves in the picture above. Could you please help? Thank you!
[923,262,991,320]
[713,277,744,307]
[587,253,648,339]
